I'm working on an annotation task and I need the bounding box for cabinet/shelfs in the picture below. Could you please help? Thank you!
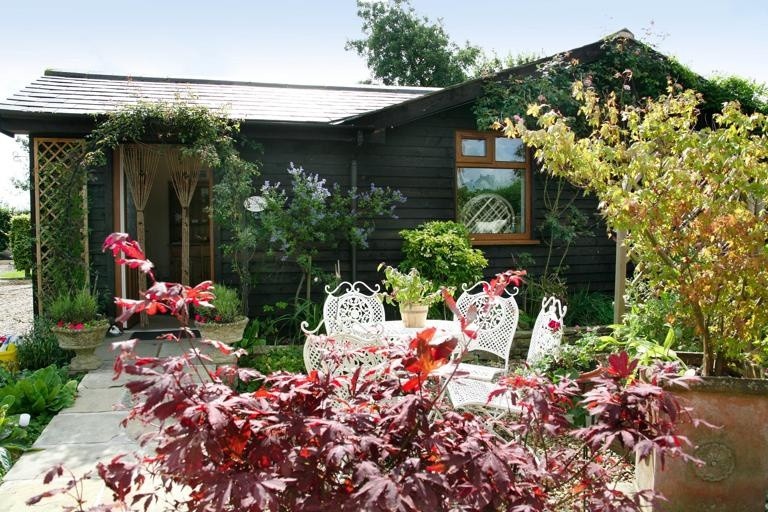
[166,179,222,285]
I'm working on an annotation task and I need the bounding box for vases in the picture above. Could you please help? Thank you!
[546,361,604,408]
[51,324,110,369]
[192,315,249,342]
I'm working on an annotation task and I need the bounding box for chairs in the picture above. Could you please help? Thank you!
[299,278,568,425]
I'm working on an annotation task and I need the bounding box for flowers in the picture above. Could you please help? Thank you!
[538,318,603,376]
[44,271,110,330]
[376,261,456,307]
[192,283,247,324]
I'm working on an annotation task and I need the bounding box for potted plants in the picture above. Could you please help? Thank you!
[493,60,768,512]
